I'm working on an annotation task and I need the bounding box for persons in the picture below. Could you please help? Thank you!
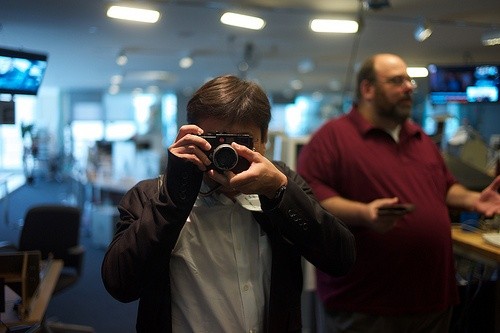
[102,75,361,333]
[297,53,499,333]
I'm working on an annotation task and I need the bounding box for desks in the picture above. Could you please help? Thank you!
[447,222,500,264]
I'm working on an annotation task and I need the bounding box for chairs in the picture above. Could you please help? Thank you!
[5,204,96,333]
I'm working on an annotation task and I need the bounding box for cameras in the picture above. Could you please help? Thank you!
[198,131,253,176]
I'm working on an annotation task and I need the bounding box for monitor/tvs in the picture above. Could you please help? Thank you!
[431,64,477,104]
[0,49,48,96]
